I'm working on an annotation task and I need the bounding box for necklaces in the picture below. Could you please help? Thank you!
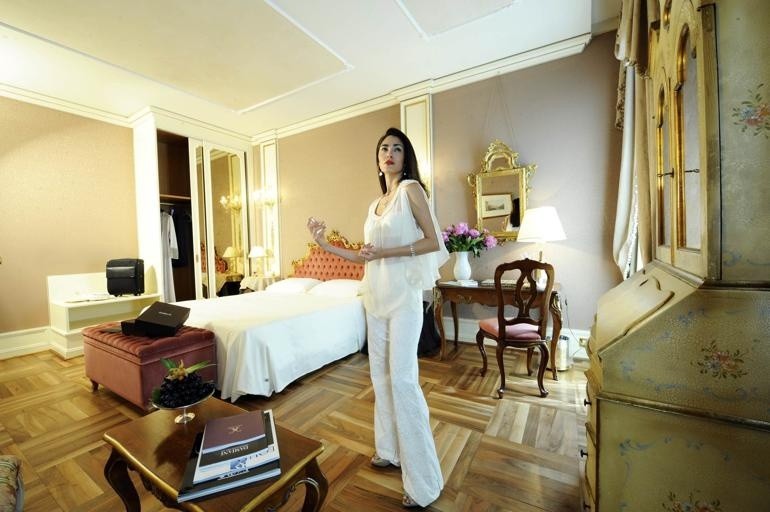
[382,193,395,207]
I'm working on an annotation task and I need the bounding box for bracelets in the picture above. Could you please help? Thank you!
[410,243,416,256]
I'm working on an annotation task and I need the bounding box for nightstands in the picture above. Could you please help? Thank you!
[202,271,241,294]
[239,276,278,293]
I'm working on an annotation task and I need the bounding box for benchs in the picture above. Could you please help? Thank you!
[82,320,216,414]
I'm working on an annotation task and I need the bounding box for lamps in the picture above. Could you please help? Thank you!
[222,247,242,272]
[251,188,278,254]
[247,246,268,276]
[518,206,567,289]
[219,192,243,248]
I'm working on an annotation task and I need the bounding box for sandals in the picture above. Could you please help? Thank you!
[402,493,417,507]
[371,456,390,467]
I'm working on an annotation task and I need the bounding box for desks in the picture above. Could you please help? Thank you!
[103,396,328,512]
[434,279,563,380]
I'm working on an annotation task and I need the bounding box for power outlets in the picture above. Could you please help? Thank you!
[579,337,587,348]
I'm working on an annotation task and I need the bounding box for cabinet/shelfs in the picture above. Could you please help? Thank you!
[47,266,161,361]
[156,128,198,302]
[581,0,769,512]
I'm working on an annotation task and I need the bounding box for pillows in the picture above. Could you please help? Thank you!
[266,277,324,295]
[309,278,362,297]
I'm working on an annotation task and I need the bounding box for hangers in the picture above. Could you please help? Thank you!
[158,205,186,224]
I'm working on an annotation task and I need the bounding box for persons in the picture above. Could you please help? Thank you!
[507,199,520,232]
[306,127,447,509]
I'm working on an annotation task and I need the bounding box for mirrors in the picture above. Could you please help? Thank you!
[466,140,539,247]
[194,145,245,300]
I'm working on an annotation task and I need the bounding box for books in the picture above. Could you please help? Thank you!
[175,407,282,503]
[480,277,528,285]
[442,279,478,285]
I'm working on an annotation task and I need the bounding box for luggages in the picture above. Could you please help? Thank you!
[106,258,144,297]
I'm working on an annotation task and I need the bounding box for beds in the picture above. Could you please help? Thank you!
[139,230,372,403]
[200,242,228,299]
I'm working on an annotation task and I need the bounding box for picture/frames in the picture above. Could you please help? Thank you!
[480,192,514,220]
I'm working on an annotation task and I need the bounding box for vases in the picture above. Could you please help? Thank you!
[453,251,472,282]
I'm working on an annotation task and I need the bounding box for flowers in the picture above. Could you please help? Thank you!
[440,222,497,259]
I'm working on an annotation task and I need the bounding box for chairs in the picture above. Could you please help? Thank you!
[475,258,554,399]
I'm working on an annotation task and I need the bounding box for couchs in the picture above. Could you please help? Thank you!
[0,456,25,512]
